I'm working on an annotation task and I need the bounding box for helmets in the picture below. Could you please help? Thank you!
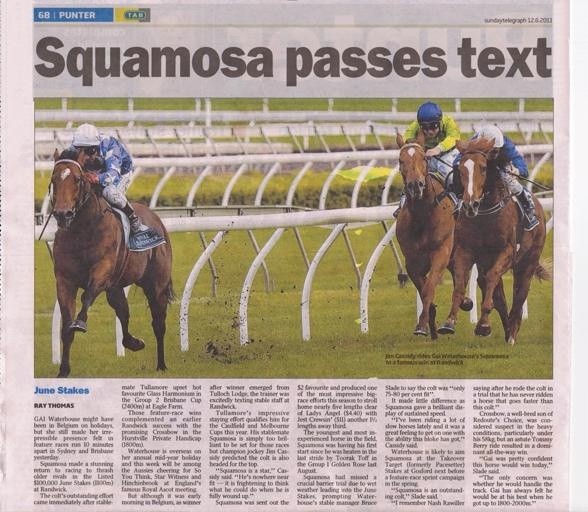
[417,102,443,123]
[478,125,504,148]
[73,124,100,148]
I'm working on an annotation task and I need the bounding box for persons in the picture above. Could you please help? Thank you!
[451,125,539,232]
[57,121,142,232]
[393,100,462,217]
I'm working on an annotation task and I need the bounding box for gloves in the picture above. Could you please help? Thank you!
[84,171,99,185]
[510,166,520,178]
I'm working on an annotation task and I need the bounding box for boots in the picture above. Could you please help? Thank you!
[122,201,140,234]
[515,186,536,226]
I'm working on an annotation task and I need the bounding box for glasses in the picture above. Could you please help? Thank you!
[419,123,440,131]
[79,148,97,155]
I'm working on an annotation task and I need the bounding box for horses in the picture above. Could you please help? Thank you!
[436,132,553,347]
[396,130,473,340]
[51,148,179,378]
